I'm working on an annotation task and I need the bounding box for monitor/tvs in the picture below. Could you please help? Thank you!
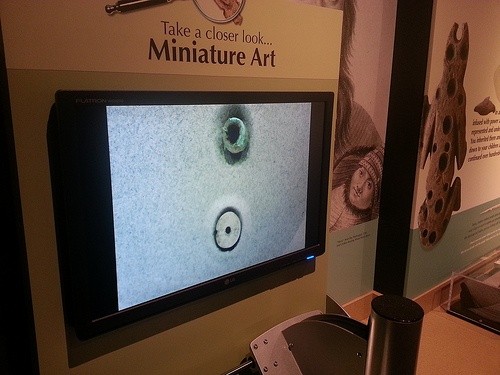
[48,86,334,342]
